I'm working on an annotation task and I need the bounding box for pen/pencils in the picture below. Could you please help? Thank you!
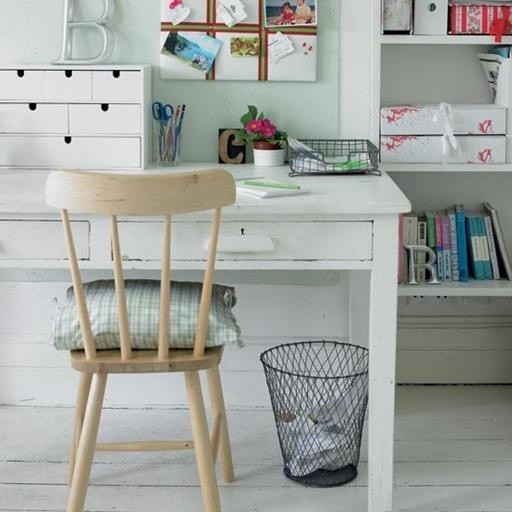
[163,104,185,161]
[244,180,301,190]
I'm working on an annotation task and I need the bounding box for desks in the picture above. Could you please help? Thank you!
[1,161,411,511]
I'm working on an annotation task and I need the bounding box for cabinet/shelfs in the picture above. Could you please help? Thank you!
[0,64,152,172]
[338,0,512,386]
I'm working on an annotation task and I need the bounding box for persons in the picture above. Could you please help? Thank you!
[280,0,312,25]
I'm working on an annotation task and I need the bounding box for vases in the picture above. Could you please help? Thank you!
[253,139,286,167]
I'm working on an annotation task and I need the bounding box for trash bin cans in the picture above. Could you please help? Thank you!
[260,341,368,488]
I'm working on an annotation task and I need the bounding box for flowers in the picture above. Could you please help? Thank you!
[233,106,288,150]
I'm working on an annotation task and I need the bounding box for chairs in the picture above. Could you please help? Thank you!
[42,170,247,512]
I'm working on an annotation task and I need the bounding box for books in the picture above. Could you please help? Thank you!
[230,177,303,199]
[398,201,512,284]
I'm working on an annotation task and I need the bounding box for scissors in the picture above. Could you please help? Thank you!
[152,102,174,163]
